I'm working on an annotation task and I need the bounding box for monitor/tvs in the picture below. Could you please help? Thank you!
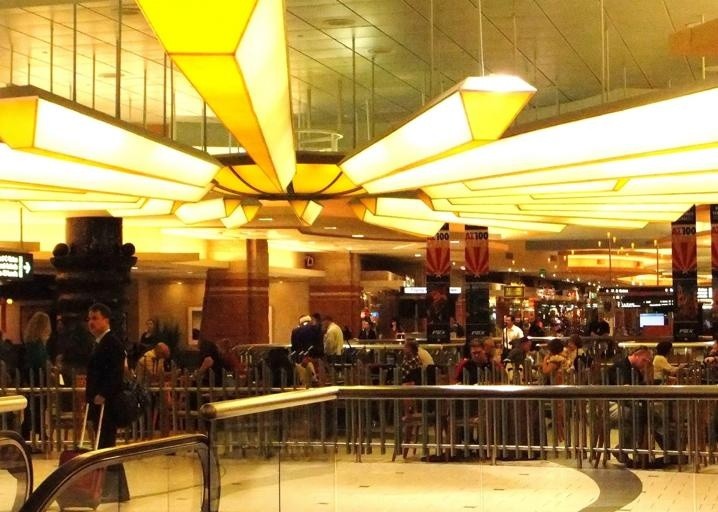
[426,324,450,344]
[638,313,665,330]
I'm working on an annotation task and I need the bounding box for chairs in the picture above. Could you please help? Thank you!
[57,361,717,463]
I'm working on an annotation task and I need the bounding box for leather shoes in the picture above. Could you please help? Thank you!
[612,448,628,462]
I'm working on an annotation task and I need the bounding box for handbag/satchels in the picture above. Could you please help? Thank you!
[111,381,151,428]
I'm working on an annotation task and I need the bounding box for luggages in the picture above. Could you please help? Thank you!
[55,398,109,511]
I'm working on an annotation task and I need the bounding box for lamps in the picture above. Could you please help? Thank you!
[0,0,718,236]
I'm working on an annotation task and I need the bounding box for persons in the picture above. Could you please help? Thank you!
[503,315,524,358]
[403,336,434,368]
[589,319,611,336]
[359,320,376,340]
[290,312,344,377]
[135,342,171,385]
[267,347,310,388]
[83,301,129,449]
[141,319,154,344]
[16,312,62,443]
[529,319,545,350]
[484,335,586,386]
[190,340,224,408]
[652,340,688,386]
[454,340,508,435]
[587,346,677,463]
[216,338,247,384]
